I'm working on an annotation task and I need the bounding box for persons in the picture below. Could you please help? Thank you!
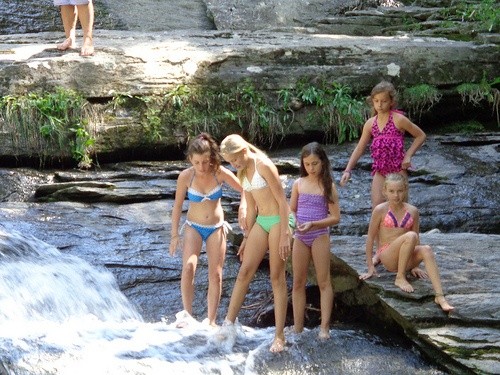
[169,133,248,328]
[220,134,296,352]
[54,0,94,56]
[285,142,340,339]
[358,174,455,311]
[340,81,426,266]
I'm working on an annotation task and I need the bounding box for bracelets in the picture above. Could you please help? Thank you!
[345,168,352,175]
